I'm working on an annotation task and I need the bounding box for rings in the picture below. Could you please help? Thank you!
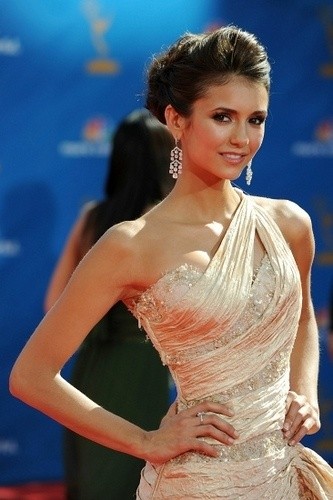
[198,411,205,424]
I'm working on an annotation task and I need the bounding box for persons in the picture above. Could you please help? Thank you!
[43,108,178,500]
[7,24,333,500]
